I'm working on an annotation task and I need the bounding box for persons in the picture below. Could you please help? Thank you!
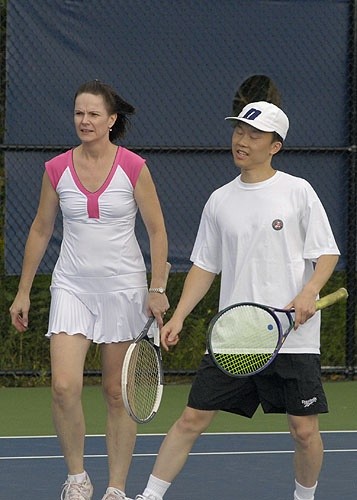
[135,101,341,500]
[9,81,171,500]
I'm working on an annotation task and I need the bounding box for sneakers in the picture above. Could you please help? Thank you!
[101,487,127,500]
[60,472,94,500]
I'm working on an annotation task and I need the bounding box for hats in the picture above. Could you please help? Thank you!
[225,99,291,141]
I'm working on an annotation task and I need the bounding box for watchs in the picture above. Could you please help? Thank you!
[149,287,166,293]
